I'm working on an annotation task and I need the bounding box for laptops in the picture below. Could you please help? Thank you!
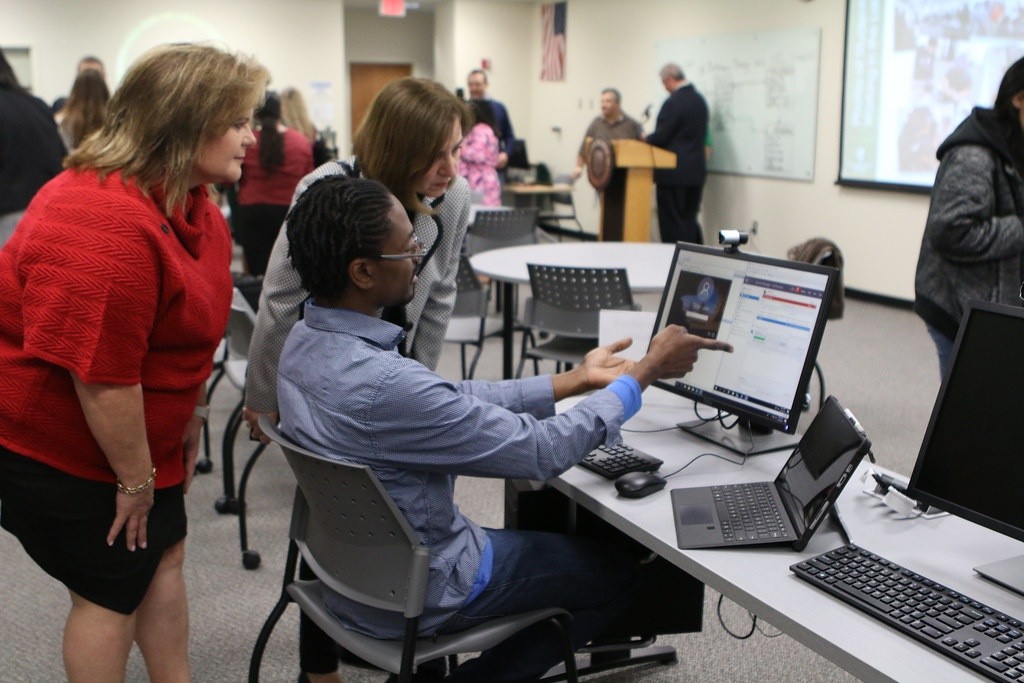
[670,396,872,552]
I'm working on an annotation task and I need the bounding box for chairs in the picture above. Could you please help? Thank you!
[788,239,845,408]
[535,166,585,242]
[197,209,644,683]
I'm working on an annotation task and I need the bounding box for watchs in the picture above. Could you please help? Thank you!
[194,406,211,421]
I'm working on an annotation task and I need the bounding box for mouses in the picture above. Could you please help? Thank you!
[614,471,669,501]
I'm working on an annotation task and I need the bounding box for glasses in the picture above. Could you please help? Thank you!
[369,232,427,263]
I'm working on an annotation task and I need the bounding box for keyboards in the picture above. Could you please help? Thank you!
[579,441,664,481]
[789,541,1024,683]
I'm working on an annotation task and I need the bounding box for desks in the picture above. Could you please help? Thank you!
[502,181,577,209]
[469,240,676,380]
[505,385,1024,683]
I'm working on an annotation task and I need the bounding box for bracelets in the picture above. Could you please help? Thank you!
[116,468,157,494]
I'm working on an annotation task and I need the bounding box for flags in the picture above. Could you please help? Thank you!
[539,2,568,82]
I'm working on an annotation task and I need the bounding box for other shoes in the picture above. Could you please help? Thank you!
[297,671,309,683]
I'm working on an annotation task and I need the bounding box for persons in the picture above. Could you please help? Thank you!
[639,62,709,245]
[276,175,734,683]
[244,76,471,683]
[456,70,516,284]
[915,56,1024,383]
[204,87,331,278]
[0,44,271,683]
[0,49,111,249]
[570,88,643,180]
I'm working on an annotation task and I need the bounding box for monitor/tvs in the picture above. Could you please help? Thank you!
[645,240,842,457]
[905,299,1024,595]
